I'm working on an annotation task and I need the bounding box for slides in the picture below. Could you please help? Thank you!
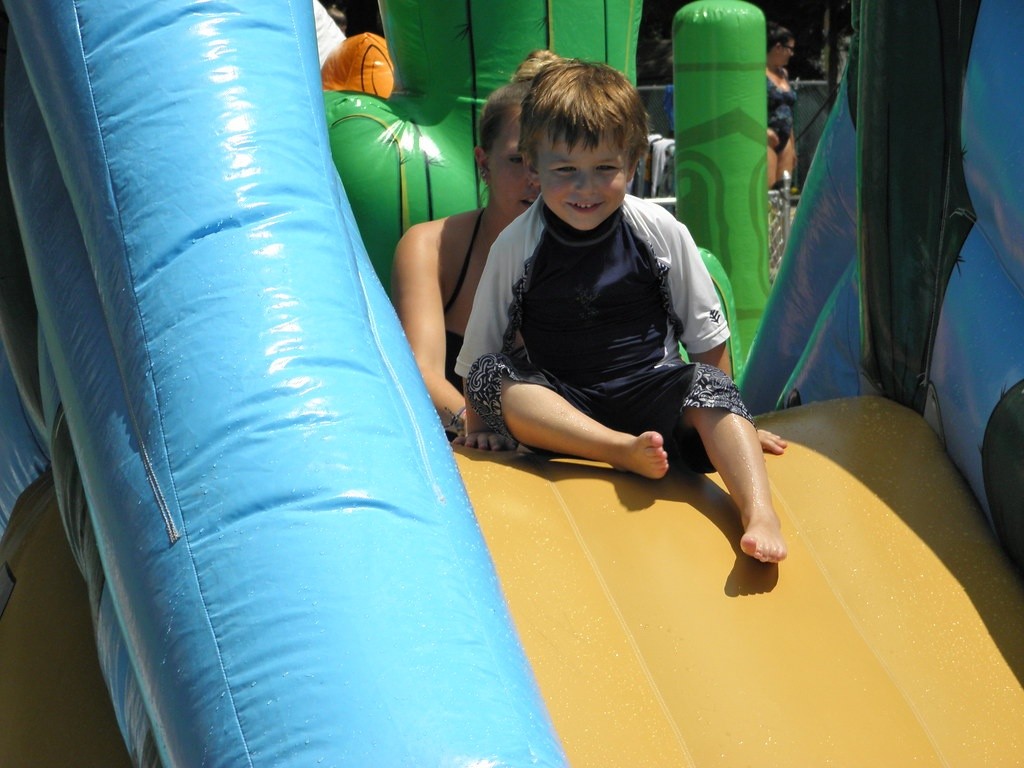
[49,387,1024,767]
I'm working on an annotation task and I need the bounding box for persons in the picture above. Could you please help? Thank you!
[457,57,787,564]
[390,48,544,451]
[764,19,796,193]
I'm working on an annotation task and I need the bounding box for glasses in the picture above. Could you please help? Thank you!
[782,44,796,52]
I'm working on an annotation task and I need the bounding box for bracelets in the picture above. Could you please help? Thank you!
[442,405,467,437]
